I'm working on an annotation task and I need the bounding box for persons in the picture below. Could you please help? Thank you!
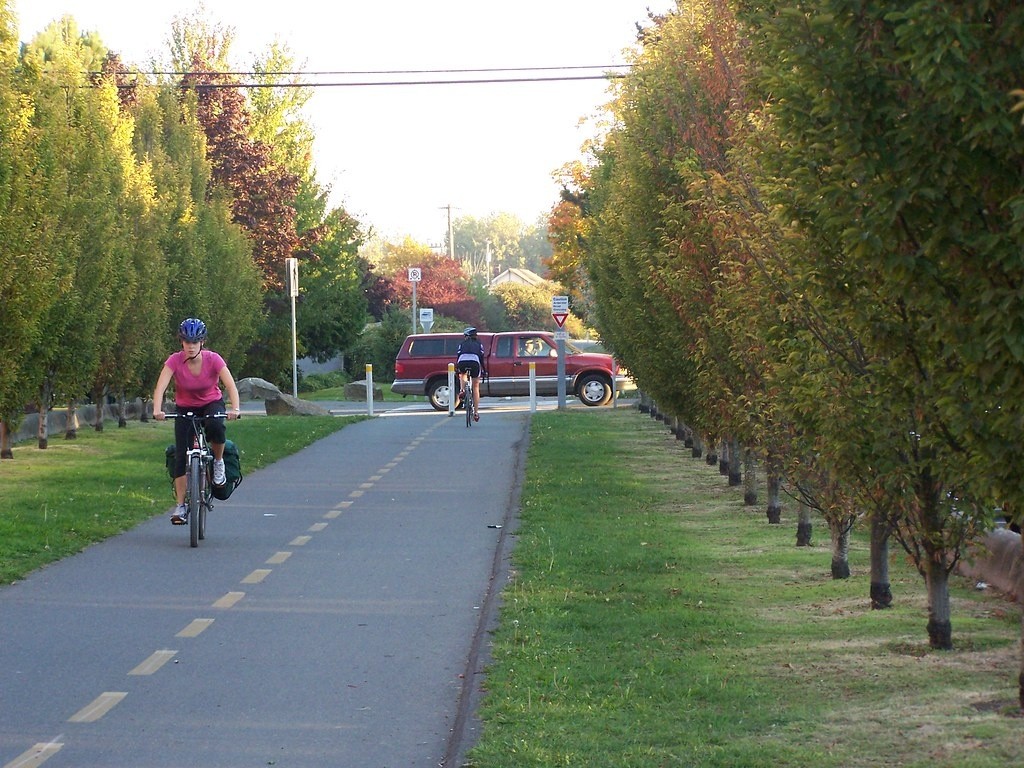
[456,327,486,422]
[153,318,240,526]
[520,338,542,356]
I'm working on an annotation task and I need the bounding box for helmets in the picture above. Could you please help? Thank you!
[464,327,476,336]
[180,318,207,342]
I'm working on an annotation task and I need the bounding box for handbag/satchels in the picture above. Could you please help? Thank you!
[208,439,243,500]
[165,444,177,478]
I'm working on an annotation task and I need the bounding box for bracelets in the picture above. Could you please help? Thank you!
[234,408,239,411]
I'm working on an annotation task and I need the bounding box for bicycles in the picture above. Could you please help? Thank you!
[152,411,241,549]
[459,366,488,428]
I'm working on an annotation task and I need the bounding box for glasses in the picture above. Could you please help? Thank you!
[464,334,466,338]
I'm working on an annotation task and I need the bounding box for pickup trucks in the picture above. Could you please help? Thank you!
[391,331,638,411]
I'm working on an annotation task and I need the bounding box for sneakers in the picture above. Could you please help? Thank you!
[170,503,189,524]
[211,457,226,487]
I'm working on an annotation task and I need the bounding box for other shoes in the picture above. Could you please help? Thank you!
[474,413,479,422]
[459,389,465,399]
